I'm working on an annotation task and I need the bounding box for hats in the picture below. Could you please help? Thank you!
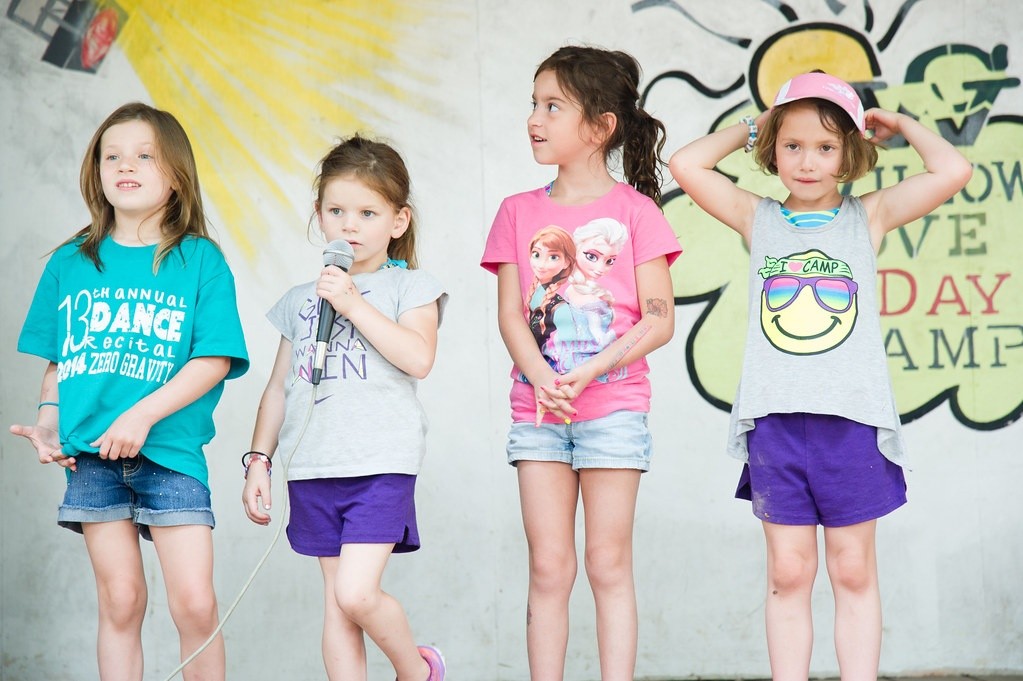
[769,73,866,140]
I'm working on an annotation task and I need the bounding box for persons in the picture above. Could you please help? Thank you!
[560,216,630,386]
[514,224,579,389]
[6,98,253,681]
[476,40,686,681]
[667,68,977,681]
[236,127,454,681]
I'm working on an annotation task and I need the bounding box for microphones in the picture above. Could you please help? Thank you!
[312,241,354,384]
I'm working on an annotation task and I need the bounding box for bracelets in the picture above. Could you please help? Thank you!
[37,401,61,412]
[241,451,272,469]
[243,454,272,481]
[737,114,759,154]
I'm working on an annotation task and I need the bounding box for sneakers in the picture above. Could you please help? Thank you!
[394,644,446,681]
